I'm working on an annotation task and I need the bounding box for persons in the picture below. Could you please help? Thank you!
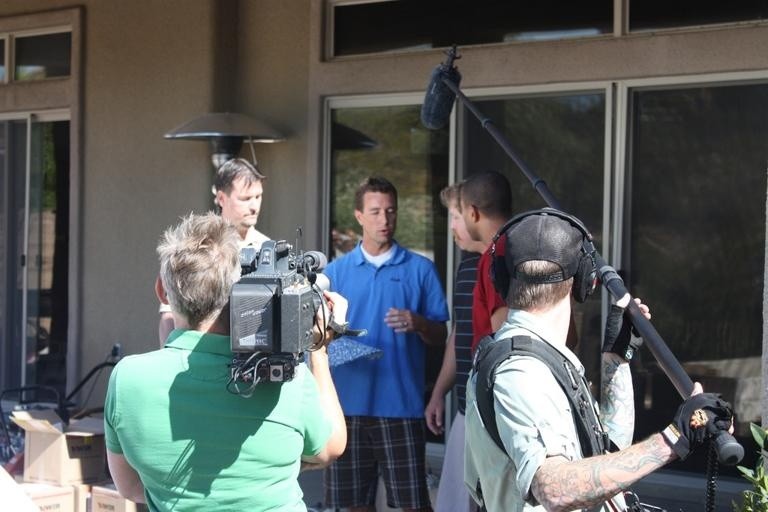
[459,173,513,353]
[321,175,450,512]
[159,156,271,349]
[424,183,488,512]
[103,211,348,512]
[463,208,734,512]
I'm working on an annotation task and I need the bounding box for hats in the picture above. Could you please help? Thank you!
[504,213,584,283]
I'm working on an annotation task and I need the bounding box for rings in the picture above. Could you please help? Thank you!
[404,321,408,327]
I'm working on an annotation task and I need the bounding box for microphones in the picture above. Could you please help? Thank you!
[421,44,462,132]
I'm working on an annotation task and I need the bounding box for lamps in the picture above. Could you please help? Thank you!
[166,113,286,168]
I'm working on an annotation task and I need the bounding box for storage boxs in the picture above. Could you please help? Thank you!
[9,407,108,485]
[91,483,149,512]
[18,483,74,512]
[70,413,105,434]
[74,480,114,512]
